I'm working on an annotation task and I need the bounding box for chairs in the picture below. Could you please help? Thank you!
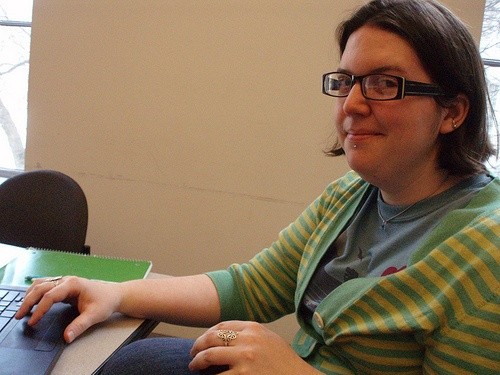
[0,170,90,255]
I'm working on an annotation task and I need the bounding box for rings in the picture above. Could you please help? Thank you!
[217,329,239,347]
[44,275,62,287]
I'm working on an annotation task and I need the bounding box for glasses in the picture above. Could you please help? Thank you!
[322,72,459,101]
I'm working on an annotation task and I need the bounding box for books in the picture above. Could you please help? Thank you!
[0,247,153,287]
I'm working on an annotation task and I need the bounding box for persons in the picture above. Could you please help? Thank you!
[16,0,500,375]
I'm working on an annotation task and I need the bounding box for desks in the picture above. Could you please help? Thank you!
[0,244,177,375]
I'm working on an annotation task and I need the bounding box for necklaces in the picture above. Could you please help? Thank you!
[377,170,450,230]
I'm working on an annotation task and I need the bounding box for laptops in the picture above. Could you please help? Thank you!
[0,284,78,375]
